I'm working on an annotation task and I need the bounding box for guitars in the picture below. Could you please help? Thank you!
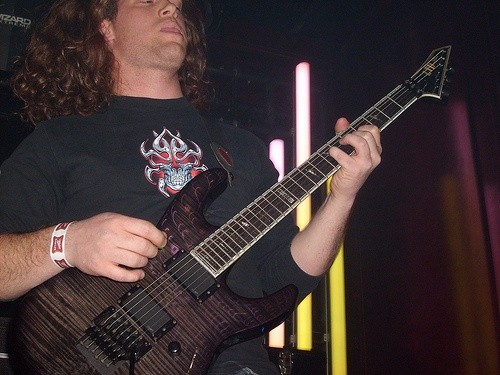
[15,45,455,375]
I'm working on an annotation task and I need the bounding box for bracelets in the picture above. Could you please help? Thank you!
[49,219,78,269]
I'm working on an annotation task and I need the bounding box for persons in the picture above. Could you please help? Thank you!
[0,0,382,374]
